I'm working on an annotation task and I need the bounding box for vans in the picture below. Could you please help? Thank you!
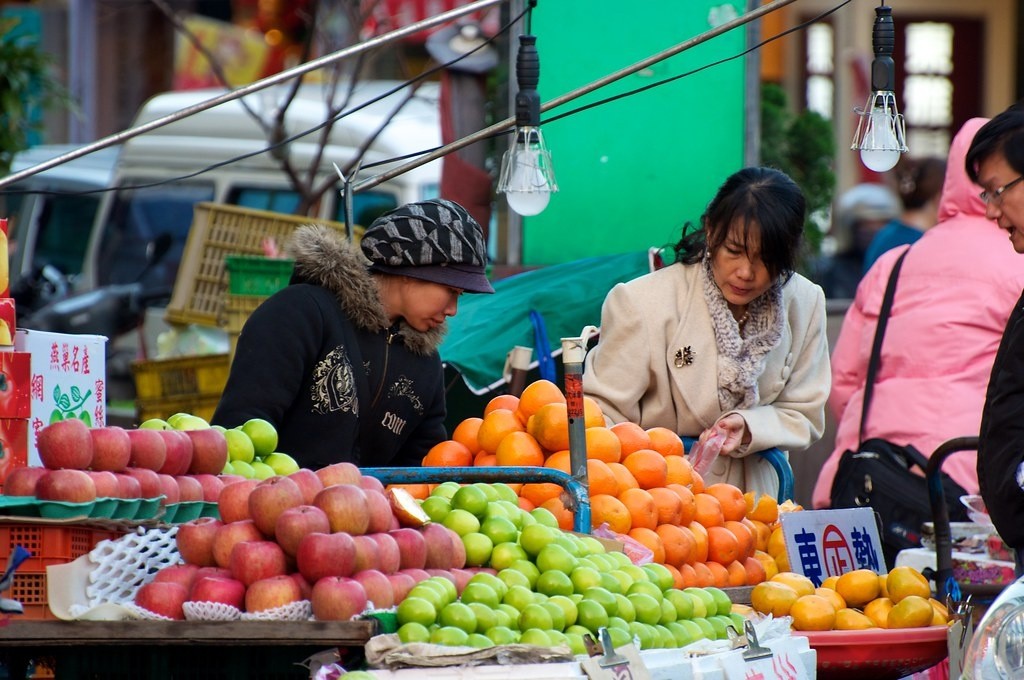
[74,92,444,297]
[9,143,208,308]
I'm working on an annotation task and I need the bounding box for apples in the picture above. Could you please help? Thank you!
[134,462,499,621]
[4,413,301,504]
[394,481,747,656]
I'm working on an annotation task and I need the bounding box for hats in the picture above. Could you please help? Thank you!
[360,198,495,294]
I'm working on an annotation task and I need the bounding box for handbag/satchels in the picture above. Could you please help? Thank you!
[830,449,970,572]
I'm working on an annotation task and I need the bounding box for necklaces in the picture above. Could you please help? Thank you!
[738,305,750,323]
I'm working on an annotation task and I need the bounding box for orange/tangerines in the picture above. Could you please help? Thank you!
[386,380,801,590]
[729,566,951,633]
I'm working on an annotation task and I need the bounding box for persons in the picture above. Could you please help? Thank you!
[811,116,1024,517]
[209,200,496,470]
[965,103,1024,583]
[809,151,950,299]
[580,165,832,506]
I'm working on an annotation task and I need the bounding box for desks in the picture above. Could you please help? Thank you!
[0,585,762,646]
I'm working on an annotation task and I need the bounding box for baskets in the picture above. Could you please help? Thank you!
[130,202,365,423]
[0,523,140,621]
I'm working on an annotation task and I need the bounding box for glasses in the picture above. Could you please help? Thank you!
[980,176,1024,207]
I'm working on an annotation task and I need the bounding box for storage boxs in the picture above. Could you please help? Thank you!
[130,353,229,425]
[0,521,127,619]
[0,216,31,496]
[226,296,266,366]
[792,625,949,680]
[225,251,292,299]
[15,326,108,468]
[162,198,369,332]
